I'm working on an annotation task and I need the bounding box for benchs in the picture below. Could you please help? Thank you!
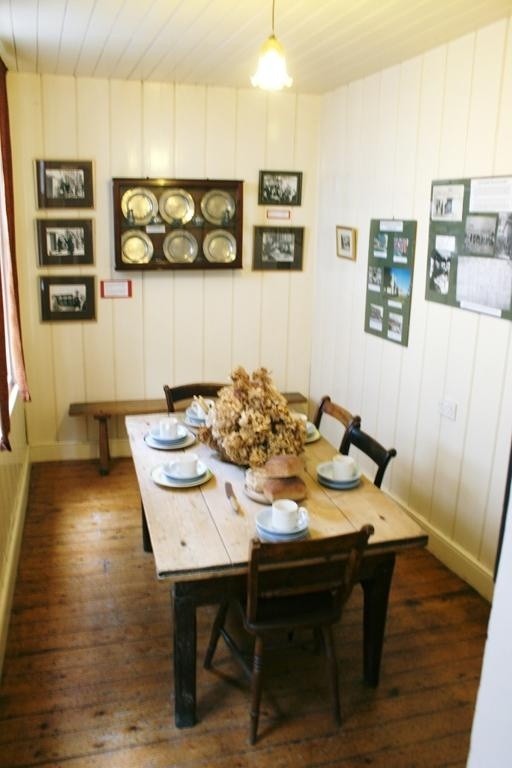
[68,392,308,474]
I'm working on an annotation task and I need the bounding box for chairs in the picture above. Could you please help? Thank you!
[205,525,375,745]
[312,395,360,452]
[163,382,231,411]
[340,422,397,488]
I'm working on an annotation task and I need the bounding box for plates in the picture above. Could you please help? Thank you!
[145,407,362,544]
[119,187,238,265]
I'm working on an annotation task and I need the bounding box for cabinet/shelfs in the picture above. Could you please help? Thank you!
[113,179,243,271]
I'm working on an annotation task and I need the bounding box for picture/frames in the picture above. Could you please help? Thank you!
[257,170,302,206]
[35,218,96,268]
[336,226,357,261]
[38,275,97,323]
[251,225,304,271]
[33,158,96,211]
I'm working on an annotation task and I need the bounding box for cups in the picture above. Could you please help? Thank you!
[160,401,357,532]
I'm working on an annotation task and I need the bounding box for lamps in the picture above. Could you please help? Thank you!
[248,0,294,93]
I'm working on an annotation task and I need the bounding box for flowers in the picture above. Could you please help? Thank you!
[194,368,306,466]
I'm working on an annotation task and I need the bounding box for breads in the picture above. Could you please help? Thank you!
[246,452,309,502]
